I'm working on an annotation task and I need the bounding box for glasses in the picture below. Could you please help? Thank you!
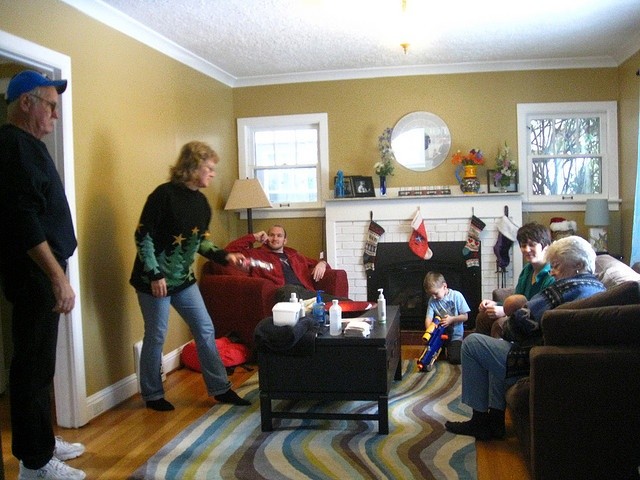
[30,94,56,111]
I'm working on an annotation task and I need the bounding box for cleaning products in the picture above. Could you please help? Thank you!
[312,289,326,326]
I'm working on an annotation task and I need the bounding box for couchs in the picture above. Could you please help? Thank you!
[200,247,350,353]
[490,253,636,478]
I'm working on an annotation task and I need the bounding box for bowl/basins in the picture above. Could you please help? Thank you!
[320,301,379,319]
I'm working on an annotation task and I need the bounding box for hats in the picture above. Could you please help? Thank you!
[6,70,67,105]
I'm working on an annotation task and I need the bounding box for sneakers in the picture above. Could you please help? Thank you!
[53,435,85,461]
[18,457,86,480]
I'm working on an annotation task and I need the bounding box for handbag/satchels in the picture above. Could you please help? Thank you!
[180,336,253,375]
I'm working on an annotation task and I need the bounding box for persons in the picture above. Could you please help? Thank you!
[128,140,253,410]
[474,221,555,341]
[357,180,371,193]
[1,71,87,480]
[224,225,353,309]
[502,293,530,318]
[418,271,464,365]
[445,235,609,440]
